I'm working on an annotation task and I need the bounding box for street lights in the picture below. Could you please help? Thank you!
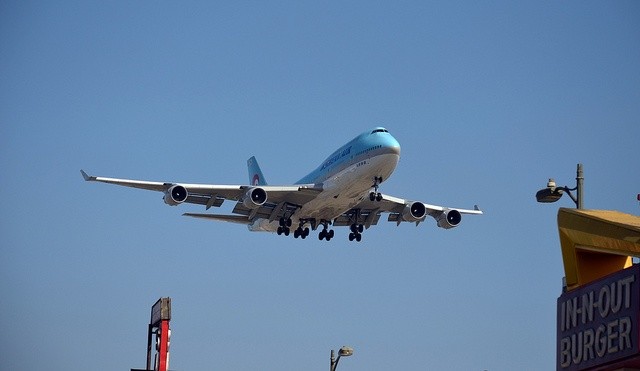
[330,344,354,370]
[534,160,585,211]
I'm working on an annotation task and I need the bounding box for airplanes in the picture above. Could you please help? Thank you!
[80,123,484,245]
